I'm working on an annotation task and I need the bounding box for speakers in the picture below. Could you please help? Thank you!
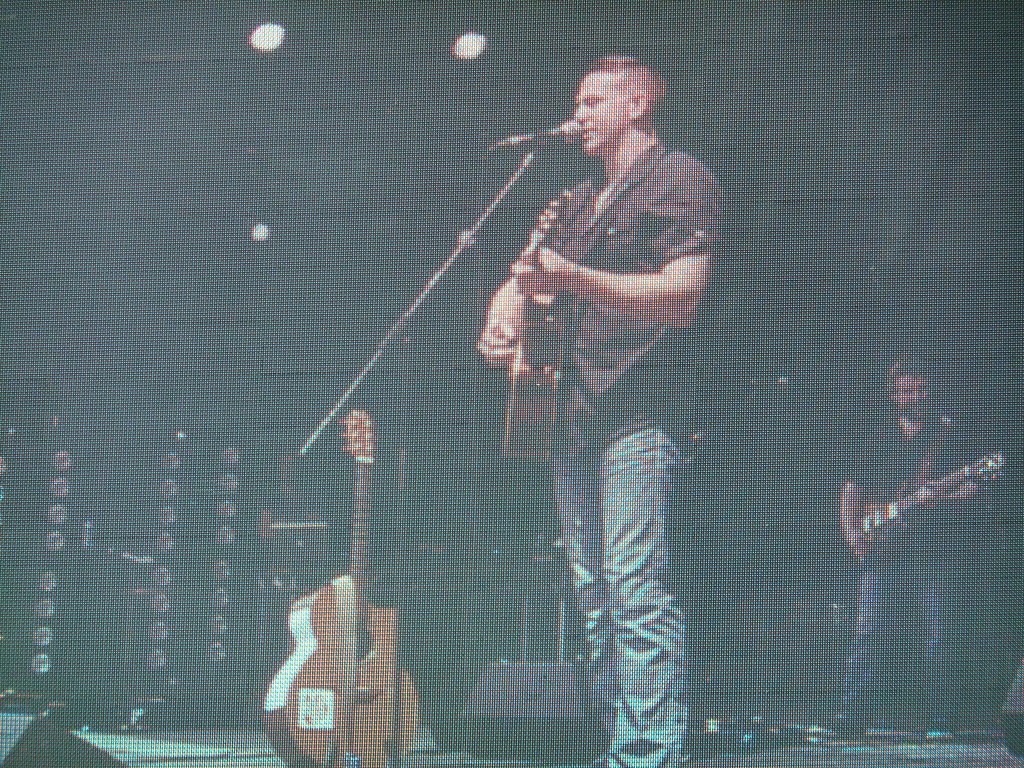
[462,660,610,761]
[1,720,129,768]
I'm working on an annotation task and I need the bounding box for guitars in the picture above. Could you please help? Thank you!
[499,185,582,464]
[845,447,1010,553]
[263,406,420,767]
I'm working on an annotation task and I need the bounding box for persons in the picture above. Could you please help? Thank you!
[476,53,722,768]
[805,349,1007,747]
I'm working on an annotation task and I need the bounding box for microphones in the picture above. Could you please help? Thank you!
[494,122,586,148]
